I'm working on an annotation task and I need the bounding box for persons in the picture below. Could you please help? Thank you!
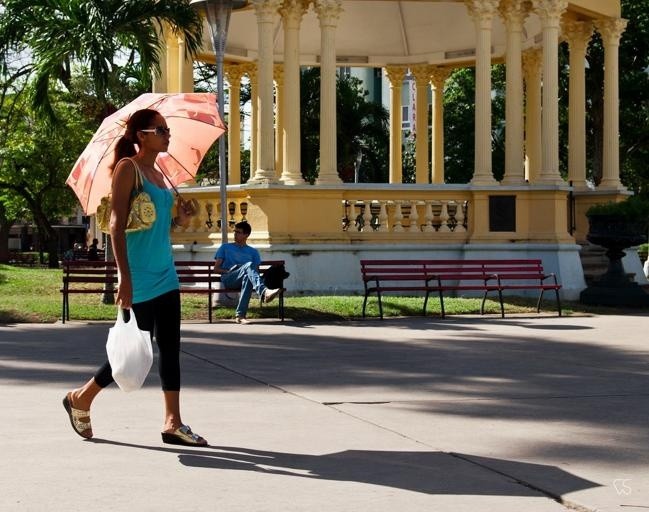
[63,109,210,447]
[88,238,105,261]
[213,221,282,324]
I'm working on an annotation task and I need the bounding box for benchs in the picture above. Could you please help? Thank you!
[59,260,287,324]
[359,259,562,323]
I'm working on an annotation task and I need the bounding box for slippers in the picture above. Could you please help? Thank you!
[63,392,92,438]
[161,425,206,446]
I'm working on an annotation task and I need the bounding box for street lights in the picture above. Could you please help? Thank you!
[189,0,251,311]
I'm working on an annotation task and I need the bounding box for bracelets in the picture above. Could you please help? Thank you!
[173,217,182,227]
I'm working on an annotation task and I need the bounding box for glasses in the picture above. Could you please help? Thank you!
[233,229,243,234]
[140,126,170,136]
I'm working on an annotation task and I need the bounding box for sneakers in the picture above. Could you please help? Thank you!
[264,288,282,303]
[235,317,251,324]
[96,191,157,234]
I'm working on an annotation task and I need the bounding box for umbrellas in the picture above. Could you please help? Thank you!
[64,92,228,216]
